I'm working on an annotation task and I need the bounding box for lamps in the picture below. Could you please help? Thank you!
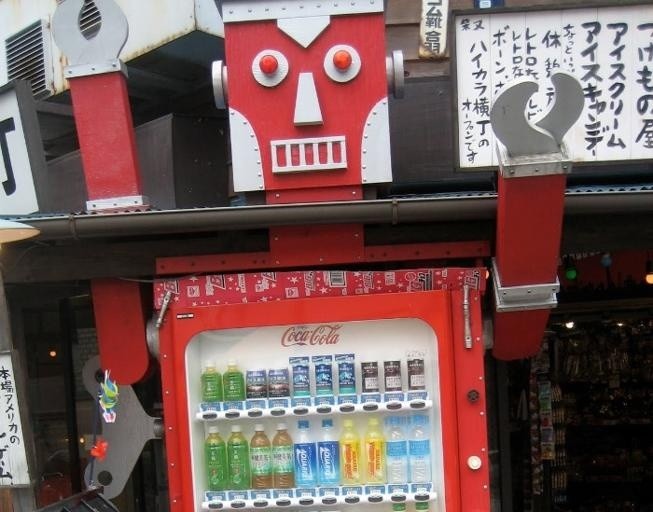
[562,255,578,280]
[597,249,612,267]
[645,257,653,289]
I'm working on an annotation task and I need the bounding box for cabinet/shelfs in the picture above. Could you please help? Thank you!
[509,369,652,505]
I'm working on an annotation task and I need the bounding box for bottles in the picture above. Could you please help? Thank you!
[386,415,407,486]
[293,419,316,488]
[202,427,227,495]
[316,420,340,488]
[339,420,364,489]
[225,425,247,492]
[222,361,245,402]
[270,423,293,493]
[200,357,222,404]
[408,418,432,485]
[247,424,271,493]
[363,416,386,487]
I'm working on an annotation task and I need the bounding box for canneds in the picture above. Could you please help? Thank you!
[268,368,289,397]
[383,361,403,392]
[361,362,378,393]
[407,358,425,391]
[245,368,267,399]
